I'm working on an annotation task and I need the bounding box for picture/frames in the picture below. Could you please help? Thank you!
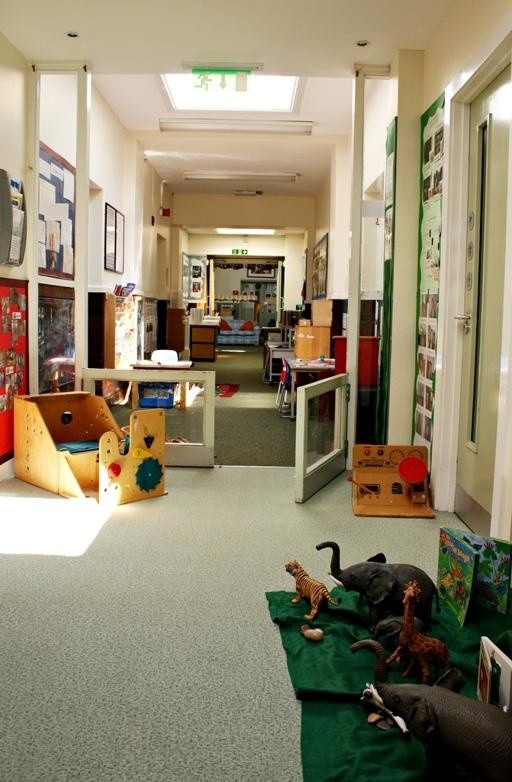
[104,202,125,274]
[311,233,328,301]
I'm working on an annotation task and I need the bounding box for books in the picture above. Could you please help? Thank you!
[189,307,222,326]
[113,282,136,297]
[436,526,512,628]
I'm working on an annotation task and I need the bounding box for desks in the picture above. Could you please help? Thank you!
[132,361,193,409]
[262,325,335,422]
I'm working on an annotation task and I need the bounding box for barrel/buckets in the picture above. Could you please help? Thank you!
[190,308,203,324]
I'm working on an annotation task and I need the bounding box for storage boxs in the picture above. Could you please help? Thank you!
[331,336,380,385]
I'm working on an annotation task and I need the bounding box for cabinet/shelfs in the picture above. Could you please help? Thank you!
[189,324,218,362]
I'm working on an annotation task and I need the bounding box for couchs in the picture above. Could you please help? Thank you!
[218,320,261,346]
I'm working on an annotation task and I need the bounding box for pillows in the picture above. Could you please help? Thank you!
[240,320,254,331]
[219,320,232,331]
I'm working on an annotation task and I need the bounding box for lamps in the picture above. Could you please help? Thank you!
[183,170,297,184]
[158,118,313,136]
